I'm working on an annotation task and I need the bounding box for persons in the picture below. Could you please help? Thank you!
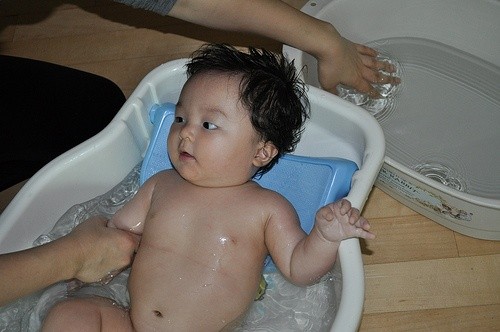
[0,0,400,303]
[40,43,376,331]
